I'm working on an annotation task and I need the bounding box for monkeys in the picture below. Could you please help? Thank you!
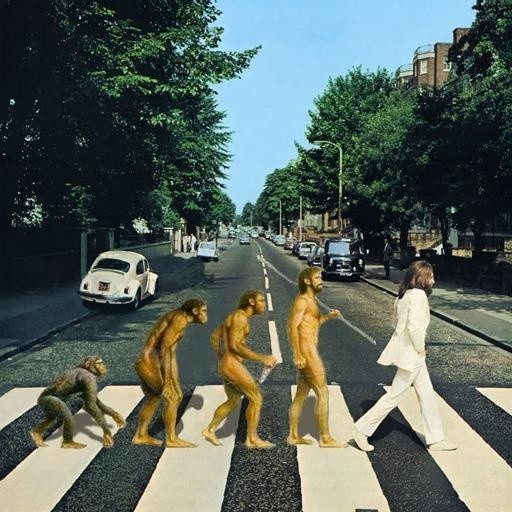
[28,355,127,449]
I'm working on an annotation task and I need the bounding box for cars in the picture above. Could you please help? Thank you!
[307,243,325,269]
[77,249,162,315]
[228,230,237,238]
[196,241,221,263]
[239,233,250,245]
[284,237,297,249]
[298,242,318,259]
[291,240,307,255]
[239,222,286,246]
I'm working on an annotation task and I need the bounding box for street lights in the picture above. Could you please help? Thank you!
[271,198,281,235]
[287,186,302,240]
[312,138,344,233]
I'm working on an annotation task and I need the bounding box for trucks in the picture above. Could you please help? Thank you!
[318,235,370,283]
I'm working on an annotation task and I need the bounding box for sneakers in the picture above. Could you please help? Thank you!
[428,439,459,451]
[350,422,374,451]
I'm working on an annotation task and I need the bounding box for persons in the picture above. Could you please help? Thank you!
[183,233,198,253]
[286,268,348,447]
[201,289,278,449]
[349,260,458,452]
[382,238,393,280]
